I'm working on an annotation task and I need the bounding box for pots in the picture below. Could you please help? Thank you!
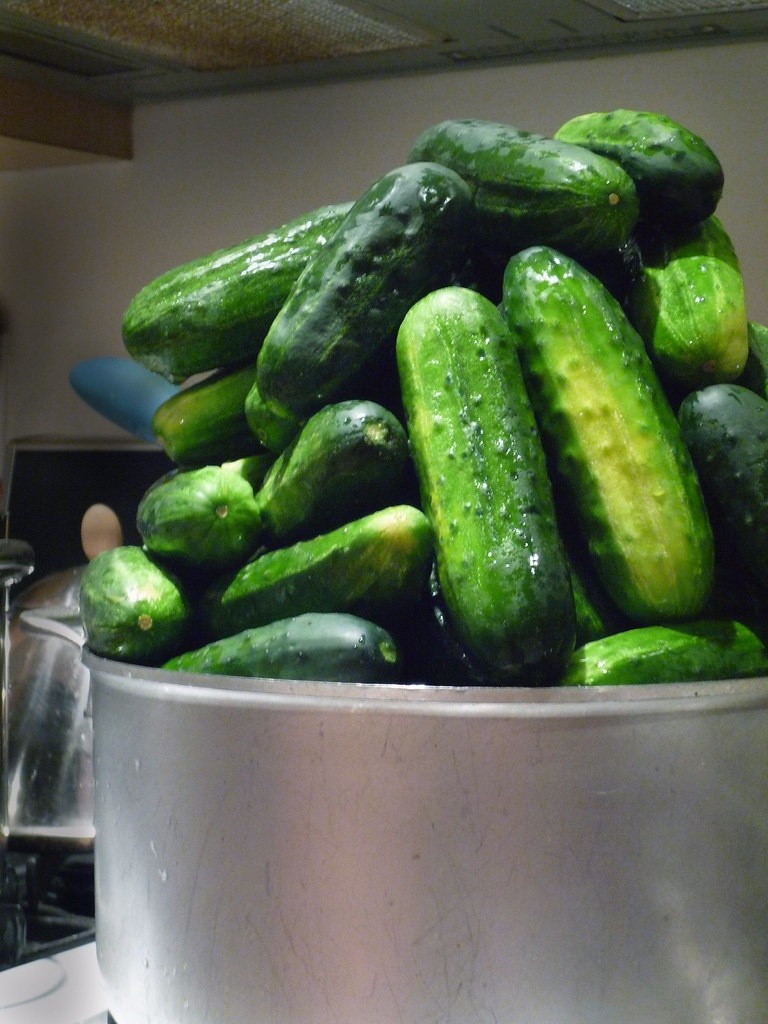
[19,607,768,1023]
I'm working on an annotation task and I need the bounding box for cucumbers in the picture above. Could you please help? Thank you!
[84,109,767,691]
